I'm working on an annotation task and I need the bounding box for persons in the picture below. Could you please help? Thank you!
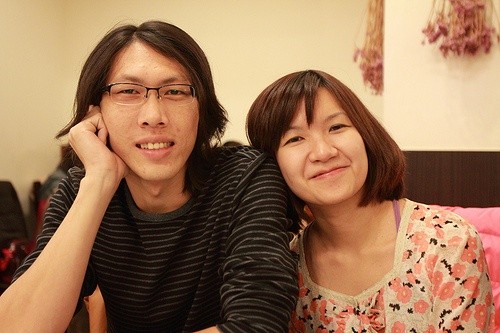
[246,68,492,333]
[0,21,299,333]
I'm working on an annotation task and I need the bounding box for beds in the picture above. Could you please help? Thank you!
[403,150,500,333]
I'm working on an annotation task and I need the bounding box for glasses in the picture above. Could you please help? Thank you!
[100,83,197,106]
